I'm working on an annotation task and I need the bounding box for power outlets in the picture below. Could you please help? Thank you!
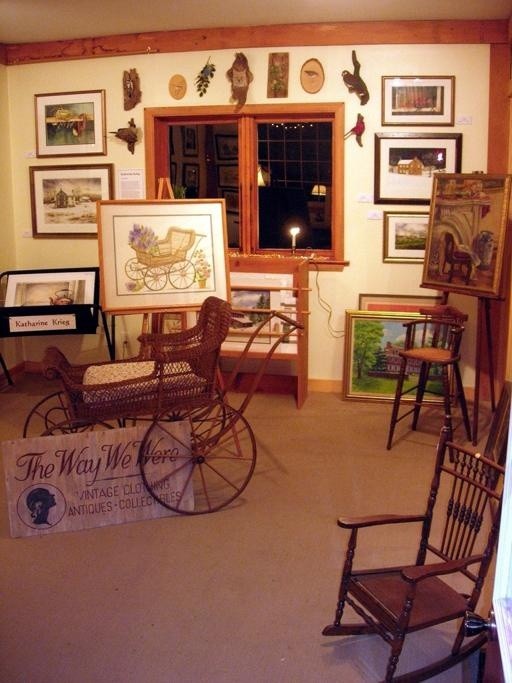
[121,332,131,349]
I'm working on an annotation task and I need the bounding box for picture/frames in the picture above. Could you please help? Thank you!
[27,164,115,240]
[381,76,456,128]
[420,173,512,300]
[482,382,511,498]
[373,132,463,206]
[342,309,454,406]
[34,89,108,157]
[187,278,282,352]
[97,198,232,311]
[170,126,238,213]
[5,267,99,320]
[358,293,443,314]
[382,211,430,264]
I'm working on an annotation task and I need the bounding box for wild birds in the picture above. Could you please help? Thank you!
[341,69,367,94]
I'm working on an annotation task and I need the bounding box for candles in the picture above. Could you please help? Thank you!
[290,227,300,246]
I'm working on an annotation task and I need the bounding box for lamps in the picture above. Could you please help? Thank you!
[311,185,327,221]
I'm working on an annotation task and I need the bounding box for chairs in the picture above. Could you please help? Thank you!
[323,415,506,683]
[386,307,471,466]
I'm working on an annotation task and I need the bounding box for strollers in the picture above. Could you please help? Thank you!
[22,297,307,521]
[127,227,206,294]
